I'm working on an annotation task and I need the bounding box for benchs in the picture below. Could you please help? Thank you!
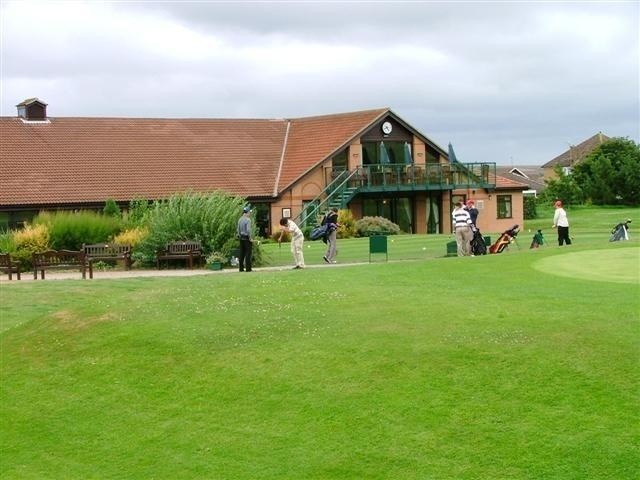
[0,239,205,282]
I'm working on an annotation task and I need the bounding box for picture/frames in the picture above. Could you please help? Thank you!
[281,207,292,218]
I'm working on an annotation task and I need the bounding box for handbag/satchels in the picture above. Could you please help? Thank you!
[608,218,633,242]
[470,227,488,256]
[529,229,546,249]
[310,222,332,241]
[489,223,521,253]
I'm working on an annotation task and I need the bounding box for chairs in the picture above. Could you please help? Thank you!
[374,165,489,186]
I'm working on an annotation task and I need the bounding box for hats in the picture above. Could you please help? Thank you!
[243,207,253,212]
[454,198,475,207]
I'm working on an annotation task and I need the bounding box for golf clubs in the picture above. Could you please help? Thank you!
[625,219,631,224]
[512,225,520,233]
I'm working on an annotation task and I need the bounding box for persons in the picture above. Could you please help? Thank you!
[236,206,254,272]
[277,218,305,269]
[451,198,478,256]
[551,200,571,245]
[321,206,339,265]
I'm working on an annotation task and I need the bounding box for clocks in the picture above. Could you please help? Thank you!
[381,120,393,136]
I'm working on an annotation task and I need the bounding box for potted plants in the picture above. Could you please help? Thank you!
[206,251,228,270]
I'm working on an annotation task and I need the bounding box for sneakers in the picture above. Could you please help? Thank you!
[322,256,337,264]
